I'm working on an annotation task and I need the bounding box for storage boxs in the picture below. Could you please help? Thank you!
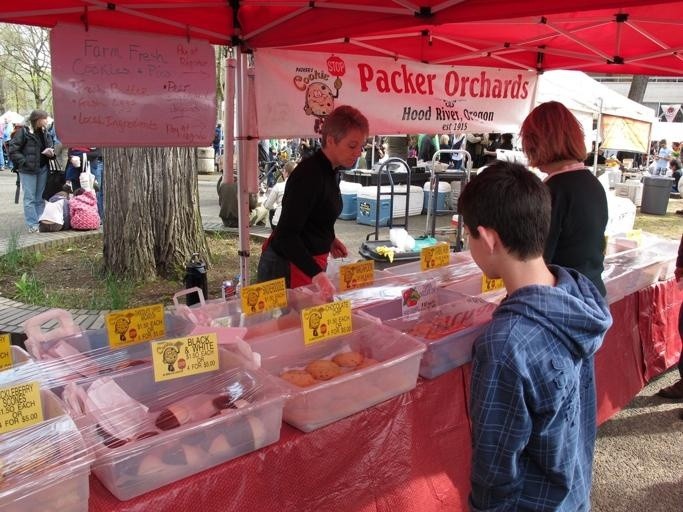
[614,249,667,292]
[646,240,680,282]
[300,270,421,312]
[601,262,634,306]
[393,187,424,218]
[36,313,197,396]
[357,186,392,227]
[62,348,291,502]
[193,288,325,351]
[337,182,362,221]
[423,181,451,217]
[220,314,427,434]
[0,342,39,389]
[0,389,92,508]
[360,287,495,381]
[384,253,483,288]
[438,275,508,307]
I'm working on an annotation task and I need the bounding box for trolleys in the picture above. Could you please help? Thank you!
[425,149,472,252]
[366,155,412,241]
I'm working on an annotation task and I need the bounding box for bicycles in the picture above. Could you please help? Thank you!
[216,160,285,193]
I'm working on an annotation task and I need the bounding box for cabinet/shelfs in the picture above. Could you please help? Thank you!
[88,275,682,512]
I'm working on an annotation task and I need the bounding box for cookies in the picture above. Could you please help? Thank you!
[306,360,340,380]
[333,352,361,367]
[281,371,315,387]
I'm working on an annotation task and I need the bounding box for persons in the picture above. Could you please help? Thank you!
[407,136,418,159]
[64,145,100,193]
[670,142,680,157]
[0,119,6,171]
[467,133,488,168]
[218,183,267,228]
[655,139,671,175]
[9,108,56,234]
[659,231,683,424]
[256,105,369,306]
[418,134,440,160]
[521,100,609,297]
[213,124,222,151]
[484,133,513,163]
[266,162,296,231]
[668,159,683,192]
[69,188,101,231]
[67,147,103,225]
[450,133,466,168]
[38,191,69,233]
[299,138,315,158]
[364,136,382,169]
[456,160,613,512]
[439,134,452,163]
[418,134,437,162]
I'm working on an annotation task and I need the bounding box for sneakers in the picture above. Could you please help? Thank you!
[657,378,683,400]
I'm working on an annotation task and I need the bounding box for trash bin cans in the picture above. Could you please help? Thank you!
[640,174,675,216]
[358,234,438,270]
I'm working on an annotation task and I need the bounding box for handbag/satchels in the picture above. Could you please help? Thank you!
[42,157,67,201]
[78,152,96,191]
